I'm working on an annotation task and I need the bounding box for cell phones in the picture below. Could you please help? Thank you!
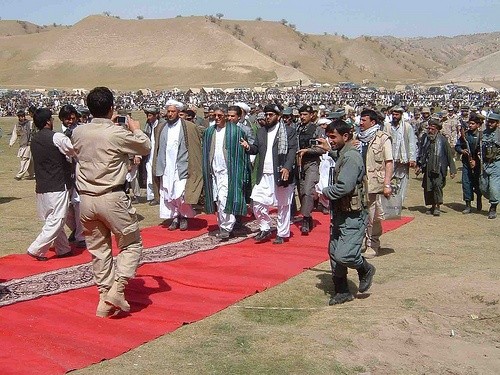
[309,140,319,145]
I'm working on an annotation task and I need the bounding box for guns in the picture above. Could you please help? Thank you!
[478,129,484,180]
[459,126,474,175]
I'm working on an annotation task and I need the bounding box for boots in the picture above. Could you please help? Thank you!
[356,256,376,294]
[488,204,498,218]
[329,278,355,305]
[462,201,471,213]
[477,195,482,210]
[96,277,130,318]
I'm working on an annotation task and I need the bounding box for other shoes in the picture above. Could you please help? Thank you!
[149,200,160,206]
[300,217,313,234]
[14,176,34,181]
[321,207,329,215]
[132,196,142,204]
[217,227,229,238]
[67,228,87,247]
[272,236,285,244]
[425,204,440,216]
[254,230,271,241]
[168,217,188,231]
[57,252,73,257]
[27,251,47,261]
[361,237,379,259]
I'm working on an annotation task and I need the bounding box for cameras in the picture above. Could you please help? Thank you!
[116,116,128,124]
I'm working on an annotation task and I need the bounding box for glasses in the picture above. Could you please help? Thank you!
[264,113,277,117]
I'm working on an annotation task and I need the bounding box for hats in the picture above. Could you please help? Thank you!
[420,105,500,130]
[233,102,319,120]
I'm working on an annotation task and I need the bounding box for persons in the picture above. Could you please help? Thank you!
[69,89,153,319]
[320,121,376,307]
[0,87,499,254]
[26,107,78,261]
